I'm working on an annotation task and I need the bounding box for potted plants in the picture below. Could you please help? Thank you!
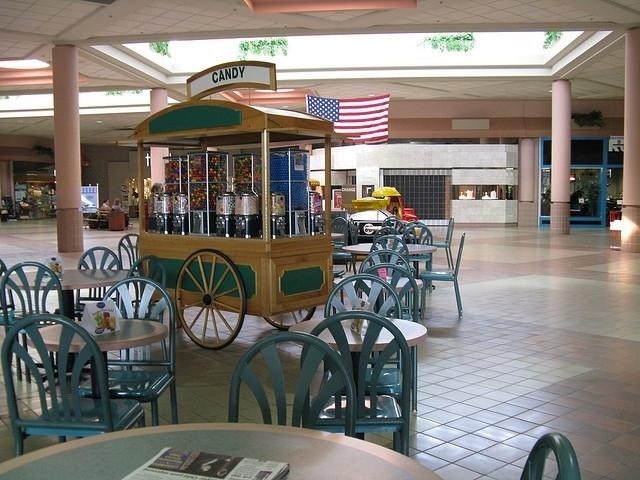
[573,107,609,131]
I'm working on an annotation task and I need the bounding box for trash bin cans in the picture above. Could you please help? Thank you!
[108,211,125,231]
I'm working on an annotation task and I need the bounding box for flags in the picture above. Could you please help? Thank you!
[306,93,391,145]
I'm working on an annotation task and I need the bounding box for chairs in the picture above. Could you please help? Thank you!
[1,228,139,379]
[101,278,178,425]
[1,314,146,460]
[333,209,467,318]
[301,310,410,460]
[325,276,417,412]
[519,430,584,480]
[228,331,355,438]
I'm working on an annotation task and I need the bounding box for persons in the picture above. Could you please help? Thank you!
[151,183,164,193]
[112,199,133,229]
[102,200,111,209]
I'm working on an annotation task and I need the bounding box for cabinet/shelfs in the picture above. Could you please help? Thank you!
[162,148,312,240]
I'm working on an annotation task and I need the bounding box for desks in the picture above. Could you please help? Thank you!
[289,316,428,441]
[2,422,442,479]
[25,303,167,405]
